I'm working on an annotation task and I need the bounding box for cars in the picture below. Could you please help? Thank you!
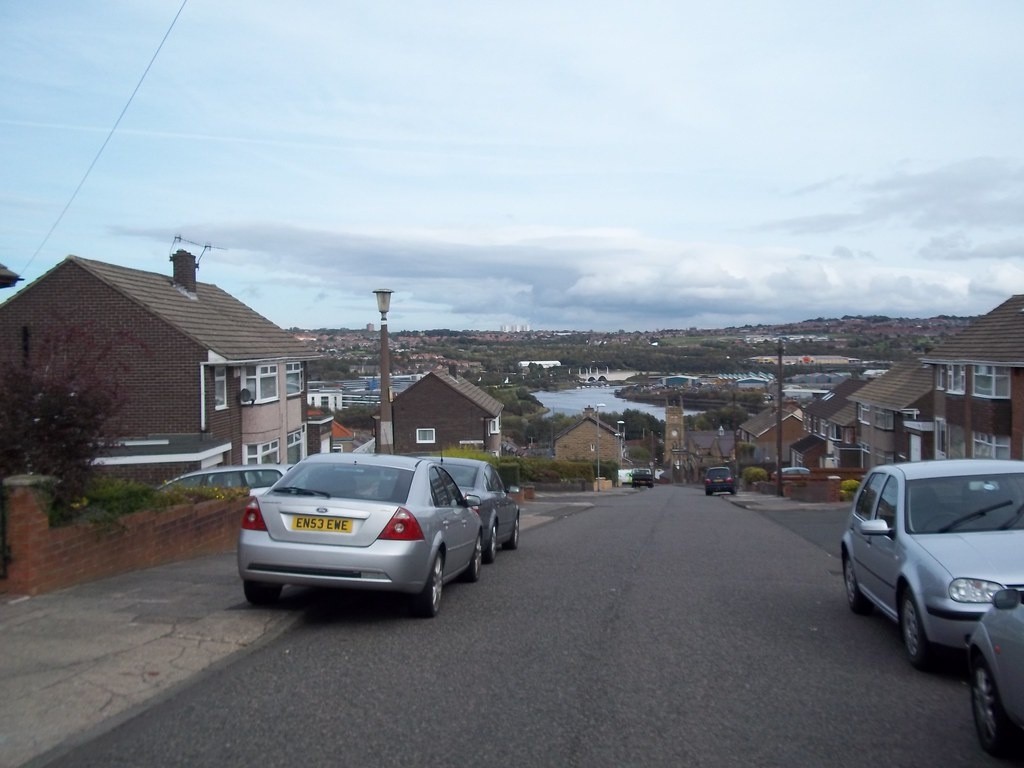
[774,467,810,474]
[967,588,1024,768]
[841,459,1024,670]
[157,464,295,497]
[236,452,484,619]
[704,466,738,496]
[632,469,654,488]
[414,456,521,565]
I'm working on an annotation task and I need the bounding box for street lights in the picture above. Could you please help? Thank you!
[372,288,395,455]
[617,421,625,487]
[596,404,606,493]
